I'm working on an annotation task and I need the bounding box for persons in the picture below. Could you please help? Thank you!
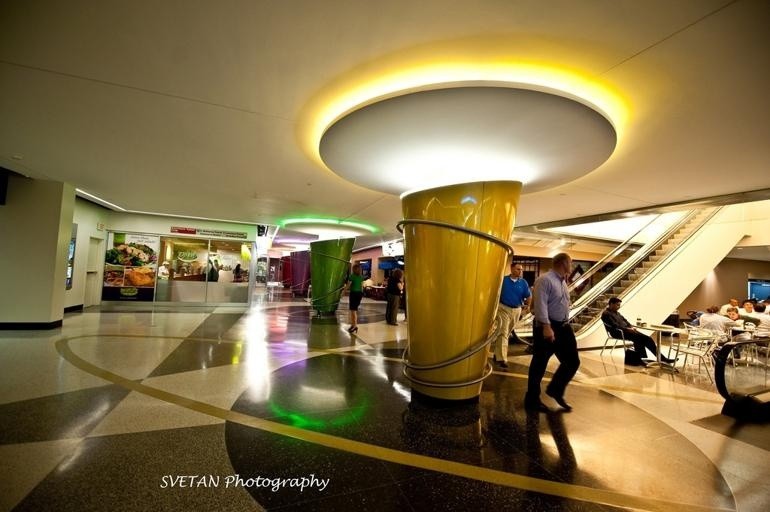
[208,259,215,281]
[744,302,770,358]
[699,305,735,366]
[343,265,364,334]
[723,307,761,359]
[493,261,533,369]
[692,311,704,327]
[602,297,679,367]
[233,264,244,283]
[386,269,405,326]
[524,252,581,413]
[739,300,759,319]
[213,260,220,281]
[720,298,739,317]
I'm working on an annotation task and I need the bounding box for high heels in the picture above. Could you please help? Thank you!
[348,326,353,331]
[350,327,357,333]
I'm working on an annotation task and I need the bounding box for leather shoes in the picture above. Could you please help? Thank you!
[499,360,508,368]
[545,389,572,411]
[524,396,549,412]
[389,322,398,325]
[665,358,679,363]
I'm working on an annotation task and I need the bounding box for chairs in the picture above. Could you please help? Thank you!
[692,328,719,374]
[730,334,769,369]
[667,329,715,383]
[682,321,701,337]
[600,322,627,358]
